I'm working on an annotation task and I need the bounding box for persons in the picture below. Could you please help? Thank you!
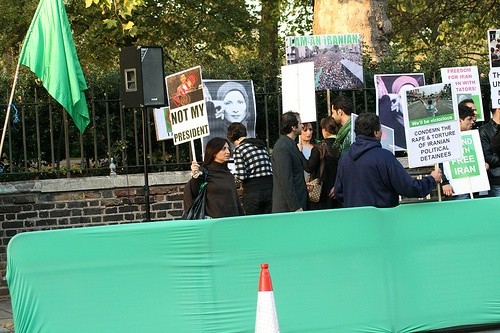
[202,82,251,137]
[271,111,310,213]
[441,98,500,201]
[416,96,441,118]
[177,73,197,106]
[378,76,420,150]
[296,97,354,211]
[226,122,275,215]
[184,135,240,219]
[334,113,440,208]
[490,37,500,67]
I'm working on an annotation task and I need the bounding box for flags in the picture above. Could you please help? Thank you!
[19,0,91,135]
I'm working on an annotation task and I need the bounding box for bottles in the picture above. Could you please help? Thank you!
[109,158,117,178]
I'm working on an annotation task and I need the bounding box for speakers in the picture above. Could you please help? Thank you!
[119,46,166,108]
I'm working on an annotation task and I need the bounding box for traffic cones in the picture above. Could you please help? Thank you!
[254,263,280,333]
